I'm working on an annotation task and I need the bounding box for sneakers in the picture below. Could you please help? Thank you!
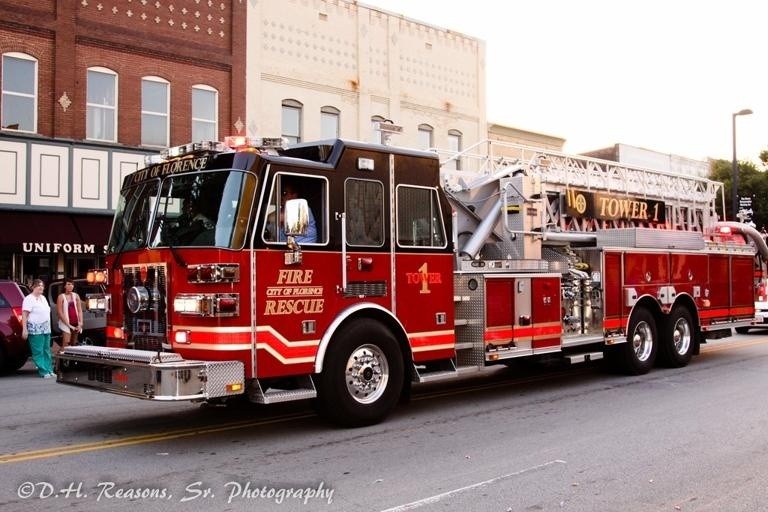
[44,373,57,379]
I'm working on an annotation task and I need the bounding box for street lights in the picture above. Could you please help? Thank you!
[731,109,753,221]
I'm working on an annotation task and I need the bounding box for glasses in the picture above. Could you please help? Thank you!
[281,189,297,197]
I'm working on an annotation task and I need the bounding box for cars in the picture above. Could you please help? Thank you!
[1,276,108,377]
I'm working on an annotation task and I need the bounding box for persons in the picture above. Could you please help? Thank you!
[266,182,318,244]
[56,278,83,351]
[21,279,58,378]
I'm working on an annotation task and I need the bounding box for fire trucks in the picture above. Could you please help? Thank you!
[54,131,762,429]
